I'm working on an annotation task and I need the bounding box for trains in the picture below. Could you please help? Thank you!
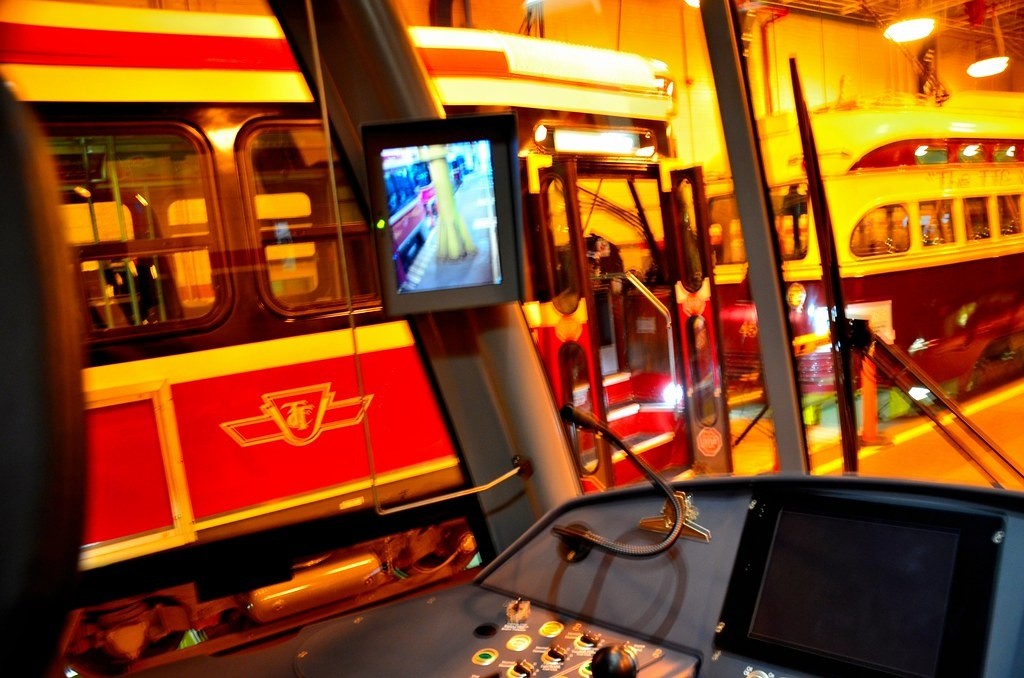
[381,148,468,262]
[0,0,711,585]
[704,91,1024,396]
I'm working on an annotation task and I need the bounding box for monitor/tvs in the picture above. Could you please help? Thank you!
[359,113,521,316]
[714,485,1002,678]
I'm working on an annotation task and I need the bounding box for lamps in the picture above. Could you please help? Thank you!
[966,41,1010,79]
[880,0,939,45]
